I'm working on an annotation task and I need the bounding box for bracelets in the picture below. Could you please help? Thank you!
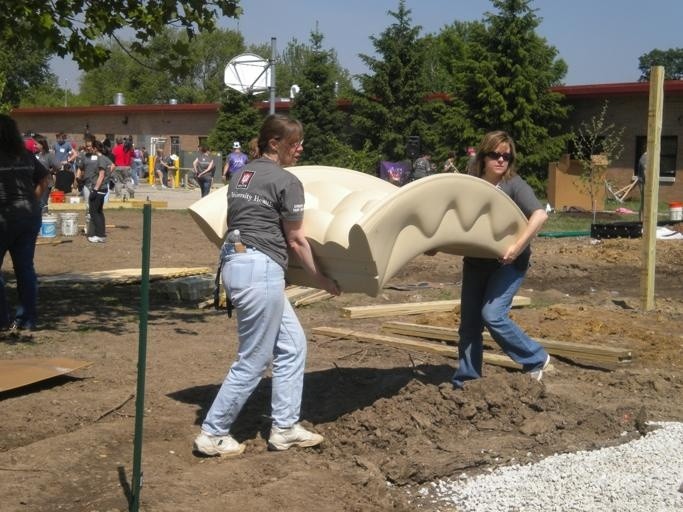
[223,174,226,176]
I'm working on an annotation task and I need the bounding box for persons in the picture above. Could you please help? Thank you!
[191,112,325,459]
[412,146,476,179]
[26,131,178,243]
[450,129,551,389]
[221,141,249,183]
[0,113,51,334]
[192,145,215,198]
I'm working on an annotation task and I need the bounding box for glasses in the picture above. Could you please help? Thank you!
[485,151,512,161]
[289,139,305,147]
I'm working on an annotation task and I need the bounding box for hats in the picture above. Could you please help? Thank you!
[170,154,178,161]
[233,142,241,149]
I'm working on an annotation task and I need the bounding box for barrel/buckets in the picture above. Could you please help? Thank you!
[69,197,80,204]
[169,98,177,105]
[112,92,124,105]
[51,191,64,203]
[668,201,683,221]
[59,212,81,236]
[38,212,57,238]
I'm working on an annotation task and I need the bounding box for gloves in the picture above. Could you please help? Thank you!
[89,189,96,201]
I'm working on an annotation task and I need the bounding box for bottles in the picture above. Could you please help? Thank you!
[225,229,241,244]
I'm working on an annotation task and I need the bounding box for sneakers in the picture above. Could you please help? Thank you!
[526,354,550,382]
[88,235,106,243]
[268,424,323,452]
[194,431,245,458]
